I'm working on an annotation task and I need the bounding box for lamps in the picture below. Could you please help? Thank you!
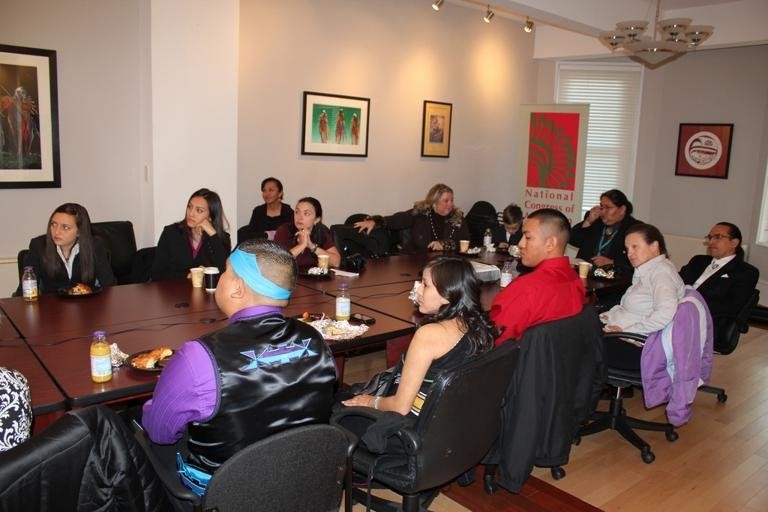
[600,1,714,70]
[432,0,444,12]
[523,16,533,33]
[483,4,495,25]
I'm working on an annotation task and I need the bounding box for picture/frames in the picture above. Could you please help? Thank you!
[421,100,452,158]
[0,44,62,189]
[674,123,734,179]
[301,91,370,157]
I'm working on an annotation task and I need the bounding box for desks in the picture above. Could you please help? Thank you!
[295,245,633,329]
[1,277,417,417]
[0,308,67,437]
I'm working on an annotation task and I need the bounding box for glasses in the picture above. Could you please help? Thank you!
[598,205,618,210]
[702,233,733,240]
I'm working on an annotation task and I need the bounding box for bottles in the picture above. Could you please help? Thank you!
[335,283,351,320]
[483,229,492,246]
[23,266,38,301]
[500,261,513,287]
[89,330,113,384]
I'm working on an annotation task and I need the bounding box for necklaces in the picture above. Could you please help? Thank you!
[429,211,455,240]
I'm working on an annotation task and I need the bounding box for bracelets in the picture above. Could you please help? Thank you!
[372,395,383,411]
[308,243,319,254]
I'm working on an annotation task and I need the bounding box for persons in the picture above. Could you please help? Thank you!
[157,188,231,280]
[13,203,118,298]
[120,241,339,510]
[233,177,295,245]
[335,256,506,456]
[0,365,34,456]
[678,222,759,342]
[598,223,686,372]
[568,190,668,308]
[274,196,341,274]
[485,208,586,348]
[354,182,466,252]
[482,203,525,252]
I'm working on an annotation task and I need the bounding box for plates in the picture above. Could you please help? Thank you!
[299,270,335,279]
[124,349,175,373]
[54,283,104,298]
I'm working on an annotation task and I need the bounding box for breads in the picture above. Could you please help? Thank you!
[131,347,173,370]
[68,283,93,295]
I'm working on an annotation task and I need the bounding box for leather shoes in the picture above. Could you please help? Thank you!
[600,385,634,400]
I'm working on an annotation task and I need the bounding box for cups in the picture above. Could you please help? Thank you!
[578,262,590,279]
[459,239,470,252]
[204,267,219,290]
[317,255,330,270]
[190,268,204,287]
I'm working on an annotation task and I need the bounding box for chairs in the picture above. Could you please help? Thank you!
[464,201,497,238]
[18,249,32,281]
[130,247,160,283]
[328,337,522,512]
[330,223,369,261]
[571,332,678,464]
[484,465,568,497]
[238,225,249,245]
[697,290,761,404]
[90,220,137,284]
[133,422,350,512]
[345,214,371,224]
[398,228,418,253]
